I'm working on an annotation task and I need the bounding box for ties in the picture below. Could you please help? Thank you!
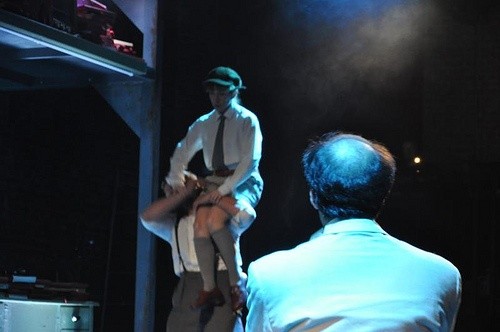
[212,116,230,177]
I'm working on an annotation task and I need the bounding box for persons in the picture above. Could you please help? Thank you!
[162,67,267,315]
[139,171,242,331]
[244,130,464,330]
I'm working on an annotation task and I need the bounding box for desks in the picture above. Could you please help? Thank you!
[0,299,99,332]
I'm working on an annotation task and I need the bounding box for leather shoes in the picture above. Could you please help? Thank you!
[190,286,225,309]
[229,272,248,315]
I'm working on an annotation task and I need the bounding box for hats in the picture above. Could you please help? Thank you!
[202,66,246,91]
